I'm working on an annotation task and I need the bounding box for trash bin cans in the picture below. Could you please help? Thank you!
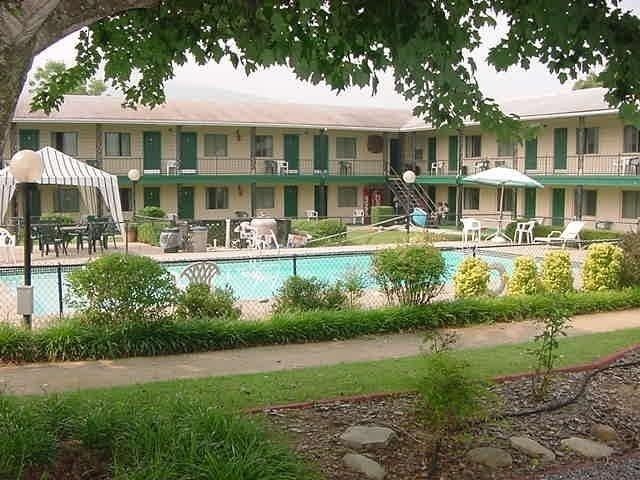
[86,160,97,168]
[274,217,292,249]
[159,226,210,254]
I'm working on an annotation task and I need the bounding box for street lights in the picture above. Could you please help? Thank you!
[127,168,141,218]
[401,170,418,244]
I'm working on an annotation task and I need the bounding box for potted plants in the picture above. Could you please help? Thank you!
[124,222,138,244]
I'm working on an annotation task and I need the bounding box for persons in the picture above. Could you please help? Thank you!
[433,201,449,225]
[430,201,443,222]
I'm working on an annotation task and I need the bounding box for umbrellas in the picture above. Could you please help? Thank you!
[461,166,544,232]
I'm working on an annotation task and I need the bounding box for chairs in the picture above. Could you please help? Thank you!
[236,216,280,250]
[338,160,354,176]
[178,262,220,290]
[272,159,288,176]
[473,158,489,174]
[303,209,319,225]
[0,227,17,265]
[431,161,447,176]
[512,220,537,246]
[534,220,586,253]
[611,157,640,176]
[459,216,481,245]
[352,207,367,224]
[27,212,120,260]
[165,160,177,176]
